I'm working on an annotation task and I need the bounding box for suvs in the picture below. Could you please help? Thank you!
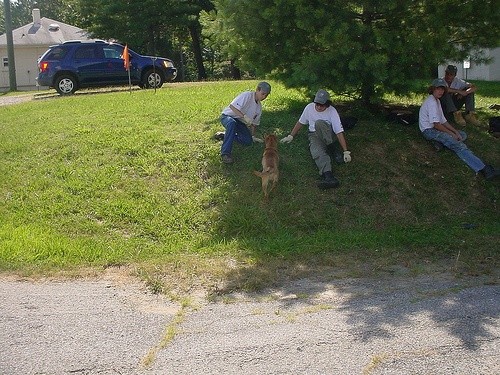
[36,39,178,96]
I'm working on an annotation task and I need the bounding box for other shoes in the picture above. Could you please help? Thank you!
[432,141,444,150]
[222,155,232,163]
[453,111,466,126]
[215,131,226,140]
[479,165,500,178]
[464,112,481,127]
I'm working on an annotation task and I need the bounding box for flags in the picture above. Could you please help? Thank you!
[124,44,129,71]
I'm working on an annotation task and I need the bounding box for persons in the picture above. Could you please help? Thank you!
[279,89,351,188]
[215,81,271,164]
[439,65,481,126]
[419,79,500,179]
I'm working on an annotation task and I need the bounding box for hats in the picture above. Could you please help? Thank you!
[314,89,329,104]
[258,82,272,94]
[445,65,457,73]
[431,79,446,89]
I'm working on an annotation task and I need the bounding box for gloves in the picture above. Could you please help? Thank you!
[241,114,252,127]
[279,135,293,144]
[252,136,264,144]
[343,151,352,163]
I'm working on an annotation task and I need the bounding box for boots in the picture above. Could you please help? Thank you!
[327,142,343,164]
[317,171,339,190]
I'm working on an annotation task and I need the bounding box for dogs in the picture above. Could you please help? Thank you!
[251,134,280,197]
